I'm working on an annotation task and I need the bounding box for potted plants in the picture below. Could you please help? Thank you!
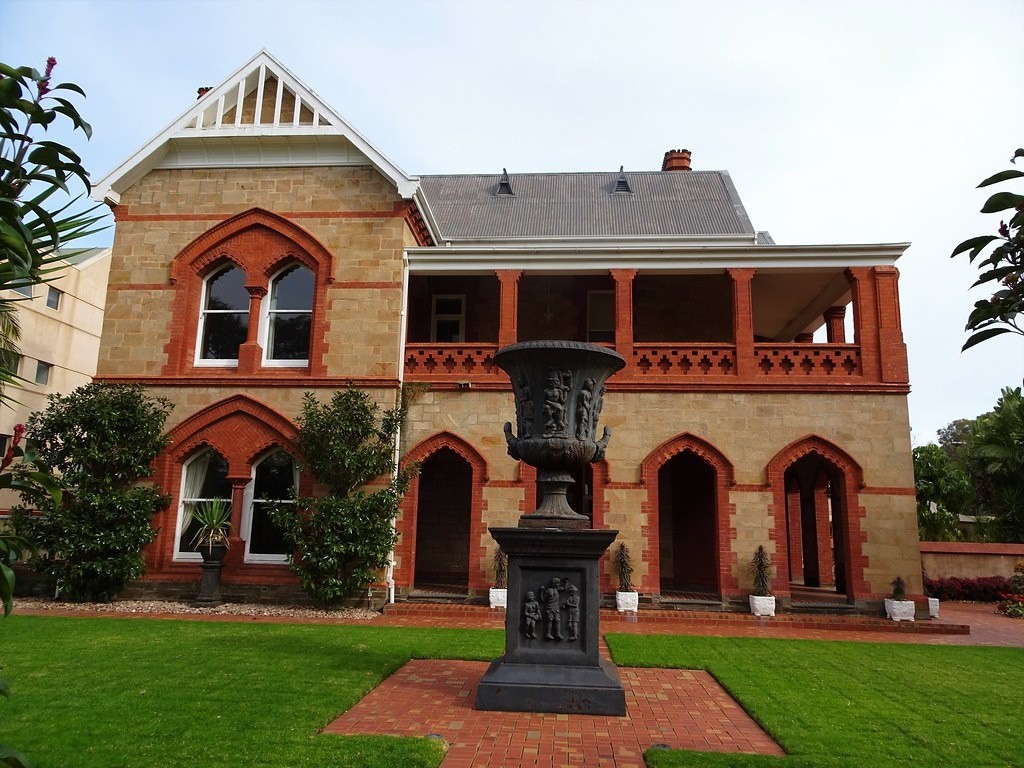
[749,544,776,616]
[884,574,916,621]
[921,559,939,617]
[188,496,238,609]
[489,545,508,608]
[613,542,639,612]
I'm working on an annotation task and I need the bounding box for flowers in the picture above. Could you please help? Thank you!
[0,423,25,473]
[928,560,1024,613]
